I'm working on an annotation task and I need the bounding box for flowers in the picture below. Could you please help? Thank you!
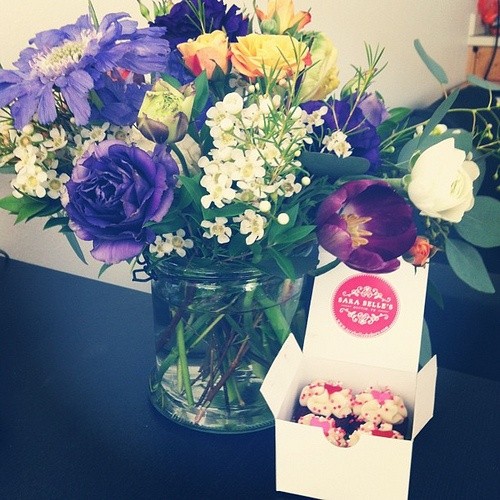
[0,0,500,428]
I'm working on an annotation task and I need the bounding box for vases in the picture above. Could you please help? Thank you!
[142,247,320,436]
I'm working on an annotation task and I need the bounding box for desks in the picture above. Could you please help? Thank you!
[0,256,500,499]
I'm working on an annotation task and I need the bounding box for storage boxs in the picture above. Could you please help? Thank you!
[258,244,440,500]
